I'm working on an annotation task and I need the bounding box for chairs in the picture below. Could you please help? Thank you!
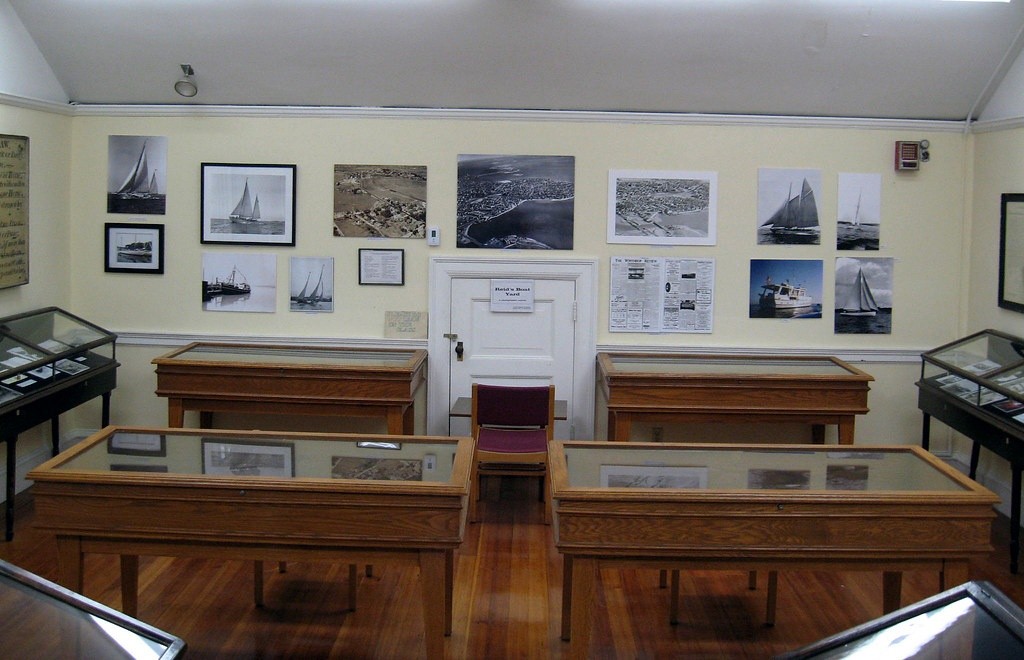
[470,382,556,525]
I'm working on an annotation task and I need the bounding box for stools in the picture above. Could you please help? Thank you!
[252,559,374,613]
[659,568,779,626]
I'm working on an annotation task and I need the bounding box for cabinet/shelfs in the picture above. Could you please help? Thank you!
[0,307,122,540]
[915,330,1024,574]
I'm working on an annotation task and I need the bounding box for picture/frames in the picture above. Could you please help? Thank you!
[200,162,296,246]
[288,256,335,313]
[201,437,297,479]
[105,222,165,274]
[328,452,425,482]
[107,431,167,457]
[998,192,1024,314]
[106,134,168,215]
[0,133,30,290]
[359,248,405,286]
[606,169,717,245]
[202,252,276,313]
[333,164,427,240]
[600,464,709,489]
[455,153,574,252]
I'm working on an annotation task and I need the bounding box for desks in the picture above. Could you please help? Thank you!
[594,351,876,445]
[25,424,477,660]
[546,444,1002,642]
[149,341,430,437]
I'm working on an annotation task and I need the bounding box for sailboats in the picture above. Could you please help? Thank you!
[228,177,263,224]
[760,175,820,240]
[844,189,866,232]
[841,265,881,319]
[295,264,328,307]
[218,264,250,294]
[113,140,160,196]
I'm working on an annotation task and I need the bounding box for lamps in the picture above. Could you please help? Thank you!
[174,63,198,98]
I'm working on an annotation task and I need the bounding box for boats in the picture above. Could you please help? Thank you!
[117,246,153,257]
[759,279,812,310]
